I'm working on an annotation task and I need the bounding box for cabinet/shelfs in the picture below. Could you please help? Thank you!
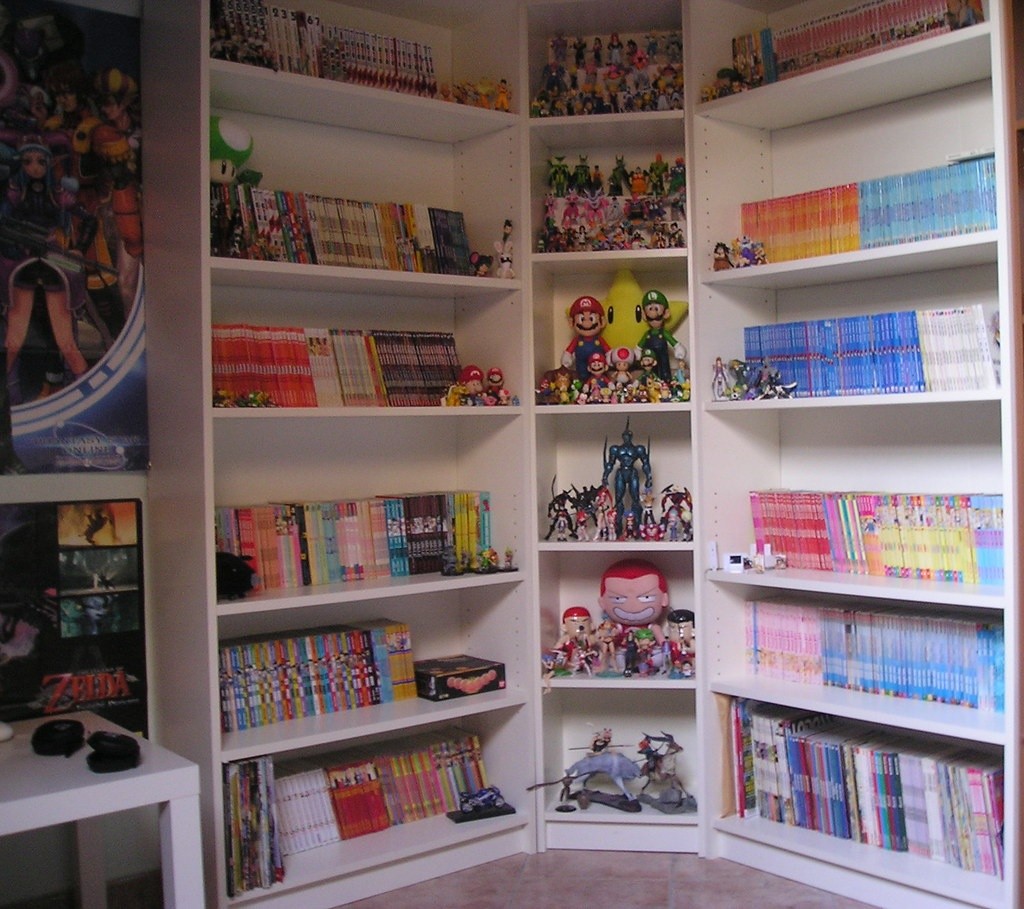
[691,0,1018,909]
[141,0,539,909]
[528,0,704,858]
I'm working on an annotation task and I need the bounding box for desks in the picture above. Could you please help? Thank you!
[0,711,205,909]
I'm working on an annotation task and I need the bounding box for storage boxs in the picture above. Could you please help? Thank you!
[413,654,507,702]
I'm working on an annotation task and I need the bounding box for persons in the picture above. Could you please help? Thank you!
[432,29,795,823]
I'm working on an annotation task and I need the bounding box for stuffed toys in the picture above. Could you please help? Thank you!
[207,114,257,183]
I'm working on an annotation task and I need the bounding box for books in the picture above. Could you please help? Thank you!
[215,490,492,592]
[744,303,996,403]
[741,157,1000,273]
[212,321,464,410]
[224,724,490,897]
[210,183,470,280]
[208,0,436,97]
[748,489,1002,594]
[730,697,1006,881]
[748,594,1004,726]
[732,0,984,94]
[215,617,419,733]
